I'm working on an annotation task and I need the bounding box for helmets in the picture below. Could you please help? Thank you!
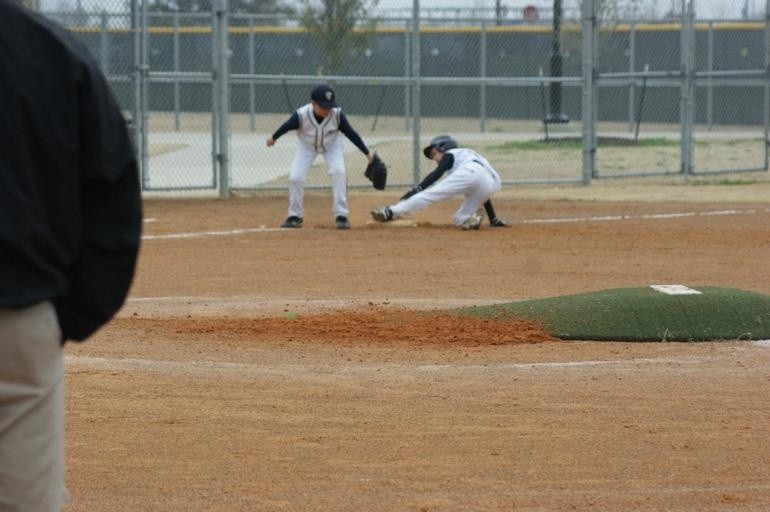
[424,136,459,159]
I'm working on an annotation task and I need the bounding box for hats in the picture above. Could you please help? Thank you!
[311,84,337,109]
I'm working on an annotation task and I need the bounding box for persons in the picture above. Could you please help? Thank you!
[370,133,511,231]
[0,0,143,512]
[265,85,374,231]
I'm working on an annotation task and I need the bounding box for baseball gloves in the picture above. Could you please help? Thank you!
[364,154,387,189]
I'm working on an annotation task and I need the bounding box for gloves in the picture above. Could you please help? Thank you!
[399,184,422,200]
[490,218,511,227]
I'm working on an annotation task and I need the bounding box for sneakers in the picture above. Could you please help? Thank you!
[335,214,349,227]
[370,206,393,222]
[281,215,303,227]
[461,216,480,231]
